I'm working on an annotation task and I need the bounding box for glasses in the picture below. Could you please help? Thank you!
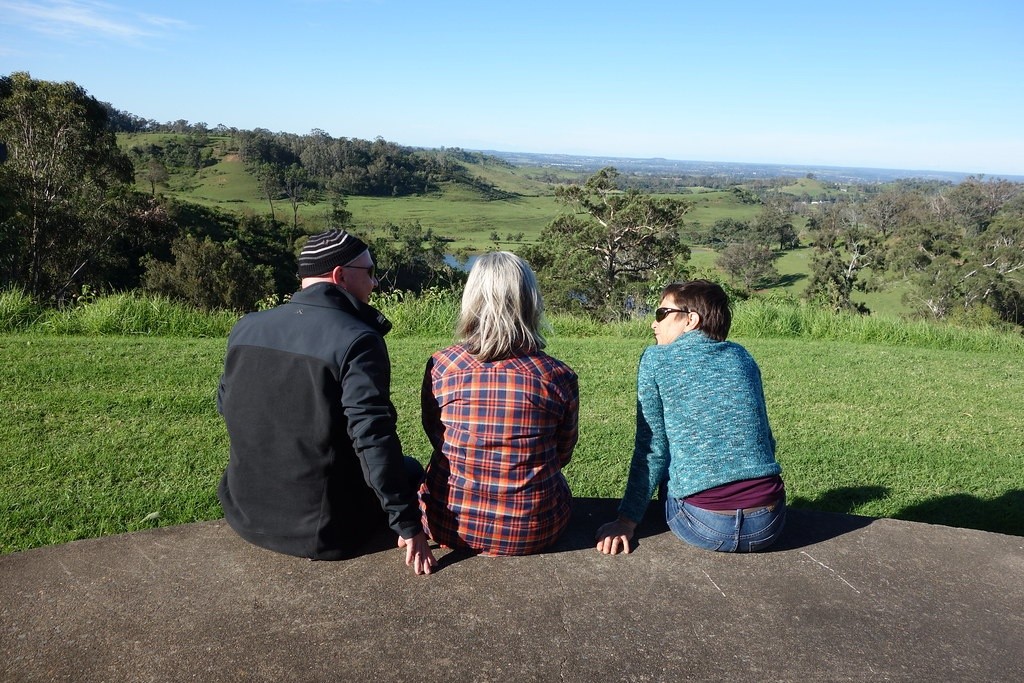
[655,307,692,323]
[340,263,376,280]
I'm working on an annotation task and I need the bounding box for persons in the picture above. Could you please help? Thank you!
[413,251,579,557]
[218,230,438,576]
[595,279,787,555]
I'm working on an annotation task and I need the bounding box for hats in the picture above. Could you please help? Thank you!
[297,228,369,279]
